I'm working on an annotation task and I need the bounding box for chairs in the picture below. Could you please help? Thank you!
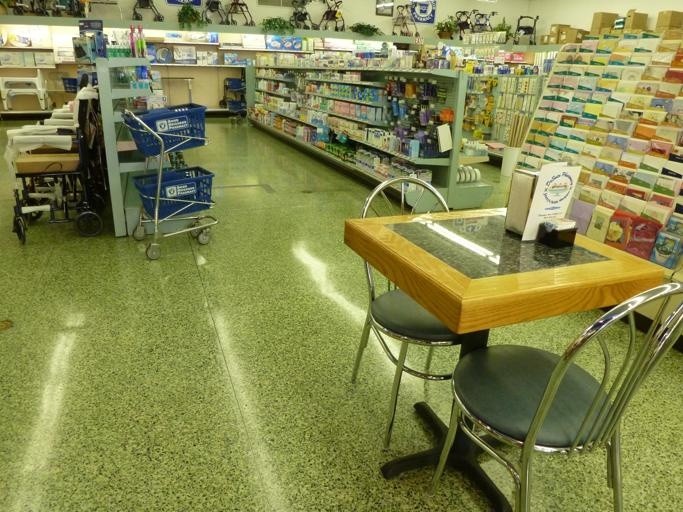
[428,282,681,512]
[352,174,460,455]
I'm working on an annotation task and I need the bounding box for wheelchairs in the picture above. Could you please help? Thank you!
[6,83,108,246]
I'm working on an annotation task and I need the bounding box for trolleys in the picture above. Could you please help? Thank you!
[114,99,221,261]
[220,77,247,116]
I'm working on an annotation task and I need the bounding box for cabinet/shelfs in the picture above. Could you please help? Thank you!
[245,59,494,210]
[97,55,198,238]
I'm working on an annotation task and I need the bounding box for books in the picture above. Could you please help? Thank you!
[504,33,683,269]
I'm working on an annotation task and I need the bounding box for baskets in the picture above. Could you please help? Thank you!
[121,101,209,158]
[130,165,216,220]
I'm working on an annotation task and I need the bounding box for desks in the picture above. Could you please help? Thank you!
[343,207,664,512]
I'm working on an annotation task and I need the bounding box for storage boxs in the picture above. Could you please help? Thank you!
[537,7,682,45]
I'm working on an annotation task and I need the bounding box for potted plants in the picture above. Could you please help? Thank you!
[434,19,456,39]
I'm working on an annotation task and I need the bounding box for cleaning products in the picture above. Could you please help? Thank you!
[304,127,311,142]
[273,115,282,128]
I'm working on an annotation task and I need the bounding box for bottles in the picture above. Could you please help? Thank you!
[102,23,148,59]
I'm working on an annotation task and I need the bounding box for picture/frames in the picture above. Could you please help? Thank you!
[375,0,393,17]
